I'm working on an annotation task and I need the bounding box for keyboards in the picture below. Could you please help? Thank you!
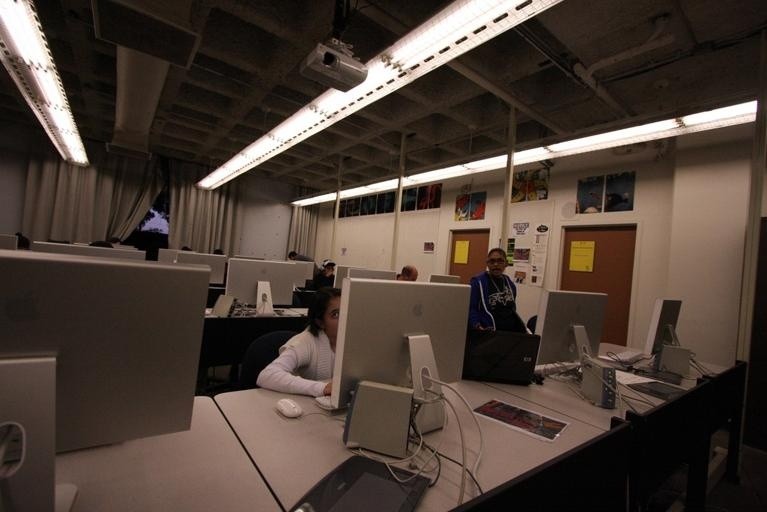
[315,395,345,410]
[611,350,642,364]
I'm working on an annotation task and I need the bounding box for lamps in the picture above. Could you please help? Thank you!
[291,101,758,206]
[2,0,90,166]
[195,1,561,190]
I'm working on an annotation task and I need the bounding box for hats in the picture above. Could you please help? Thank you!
[322,259,335,267]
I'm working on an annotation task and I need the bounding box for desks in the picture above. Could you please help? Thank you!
[0,394,287,512]
[213,382,609,512]
[204,306,303,318]
[475,341,748,511]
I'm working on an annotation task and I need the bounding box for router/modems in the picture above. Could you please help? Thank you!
[580,357,616,409]
[661,346,691,378]
[341,379,414,460]
[212,295,237,318]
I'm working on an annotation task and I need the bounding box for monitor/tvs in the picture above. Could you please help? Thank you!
[0,234,17,250]
[428,274,461,283]
[306,262,314,280]
[0,248,211,512]
[330,278,472,438]
[158,249,177,263]
[333,265,355,288]
[633,298,683,385]
[536,289,608,383]
[31,240,146,261]
[176,251,226,285]
[347,268,397,280]
[226,258,297,318]
[294,260,306,289]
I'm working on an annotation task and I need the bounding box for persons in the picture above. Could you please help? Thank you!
[112,237,121,244]
[181,246,192,251]
[15,232,30,250]
[89,241,113,248]
[314,258,336,288]
[214,249,223,254]
[396,265,418,281]
[469,248,527,333]
[289,251,314,262]
[257,289,341,397]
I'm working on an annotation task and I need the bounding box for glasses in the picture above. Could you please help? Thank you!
[485,258,505,264]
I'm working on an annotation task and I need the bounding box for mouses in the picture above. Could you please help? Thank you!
[277,398,302,418]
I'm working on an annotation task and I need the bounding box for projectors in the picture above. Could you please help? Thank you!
[298,44,368,94]
